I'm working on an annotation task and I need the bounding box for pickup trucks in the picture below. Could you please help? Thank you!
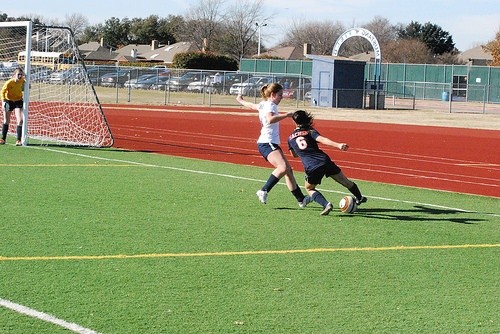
[101,68,155,88]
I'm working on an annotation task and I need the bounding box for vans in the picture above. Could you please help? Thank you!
[72,69,117,84]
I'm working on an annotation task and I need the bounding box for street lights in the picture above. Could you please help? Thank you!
[255,22,268,57]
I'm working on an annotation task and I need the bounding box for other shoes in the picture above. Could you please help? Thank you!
[16,139,22,146]
[0,139,5,144]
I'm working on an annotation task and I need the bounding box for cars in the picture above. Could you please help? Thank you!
[138,76,171,90]
[188,75,227,93]
[124,74,157,89]
[282,82,312,100]
[152,80,167,91]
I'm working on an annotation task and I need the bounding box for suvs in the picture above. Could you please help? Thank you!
[229,76,276,97]
[210,73,253,95]
[52,67,81,86]
[166,71,214,93]
[260,75,312,98]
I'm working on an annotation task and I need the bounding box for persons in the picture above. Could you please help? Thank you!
[236,83,314,207]
[0,68,31,146]
[287,110,368,216]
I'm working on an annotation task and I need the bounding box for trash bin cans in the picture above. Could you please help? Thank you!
[442,92,453,102]
[369,94,385,108]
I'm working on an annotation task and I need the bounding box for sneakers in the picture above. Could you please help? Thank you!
[256,189,268,205]
[355,196,367,205]
[298,195,313,207]
[321,202,333,215]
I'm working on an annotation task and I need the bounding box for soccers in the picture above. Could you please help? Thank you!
[340,196,357,213]
